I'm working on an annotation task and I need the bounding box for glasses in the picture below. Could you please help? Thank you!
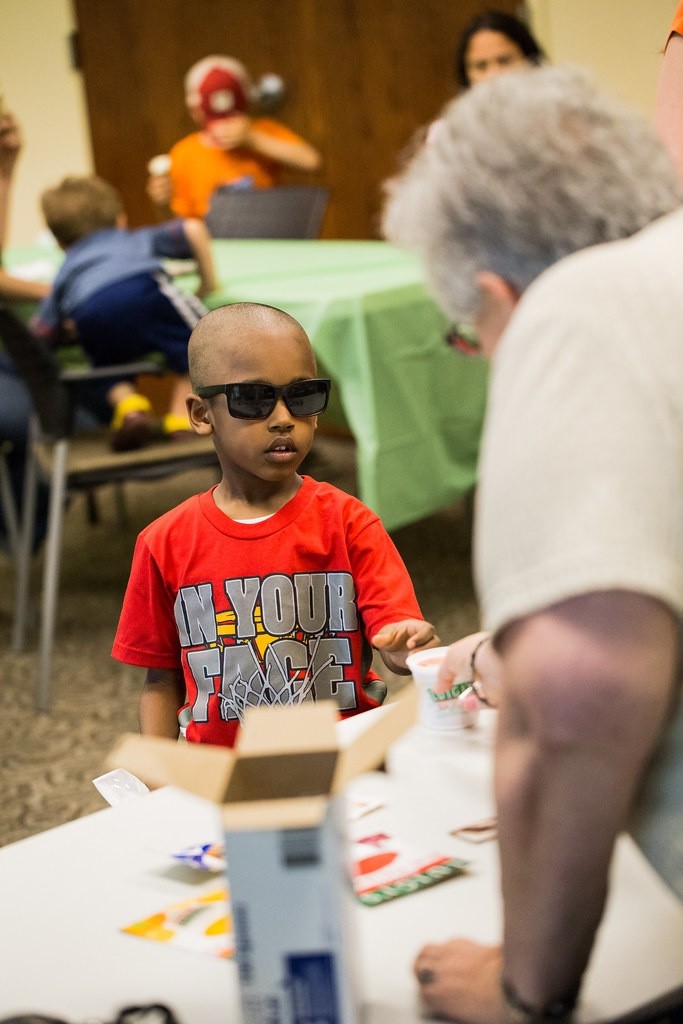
[196,378,331,421]
[444,324,481,358]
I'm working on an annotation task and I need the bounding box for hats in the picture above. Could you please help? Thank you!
[184,53,260,115]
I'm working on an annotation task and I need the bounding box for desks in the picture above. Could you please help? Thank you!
[3,239,497,540]
[0,687,683,1024]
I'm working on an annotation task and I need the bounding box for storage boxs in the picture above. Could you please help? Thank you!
[100,705,415,1024]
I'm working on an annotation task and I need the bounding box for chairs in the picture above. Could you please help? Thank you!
[0,307,222,711]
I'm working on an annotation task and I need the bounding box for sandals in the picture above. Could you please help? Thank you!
[111,392,154,453]
[164,413,196,441]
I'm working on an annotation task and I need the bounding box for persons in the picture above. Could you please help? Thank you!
[656,0,683,184]
[381,65,683,1023]
[452,10,547,91]
[26,177,217,446]
[109,300,442,794]
[0,97,53,556]
[144,56,320,219]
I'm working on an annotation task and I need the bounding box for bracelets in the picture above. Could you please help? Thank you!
[498,970,538,1019]
[468,638,497,710]
